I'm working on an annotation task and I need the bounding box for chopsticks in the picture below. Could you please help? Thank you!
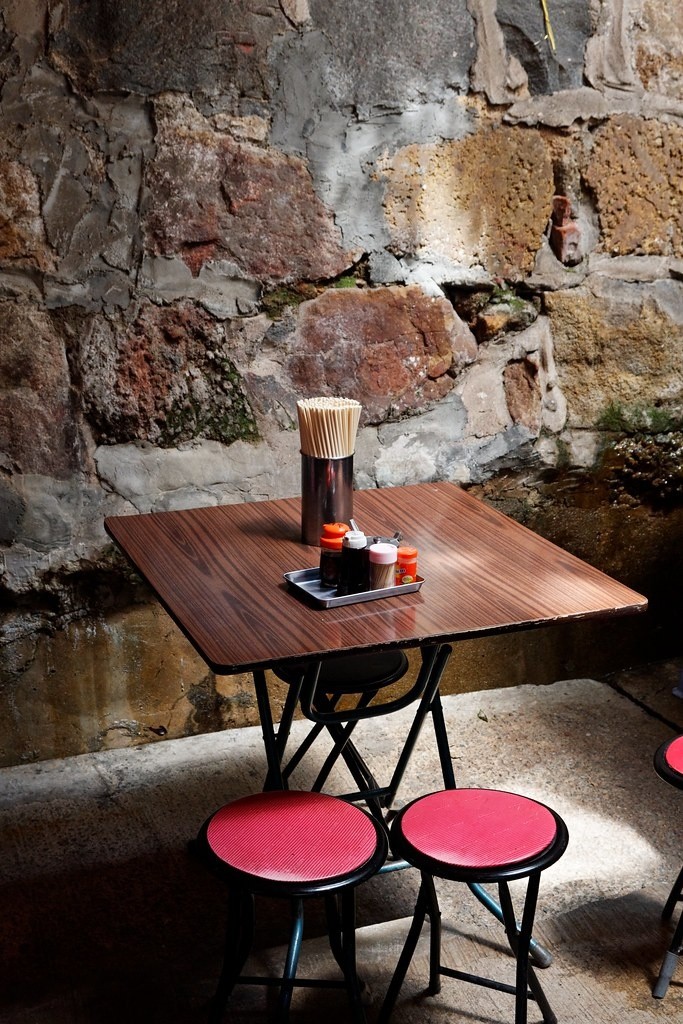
[297,397,363,458]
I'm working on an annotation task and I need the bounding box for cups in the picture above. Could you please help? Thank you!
[298,450,356,546]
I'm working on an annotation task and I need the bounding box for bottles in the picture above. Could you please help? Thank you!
[395,547,418,586]
[319,522,351,588]
[370,543,397,590]
[338,531,370,595]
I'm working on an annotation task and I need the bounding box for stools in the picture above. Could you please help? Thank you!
[373,788,569,1024]
[186,787,387,1024]
[267,650,410,792]
[653,730,683,997]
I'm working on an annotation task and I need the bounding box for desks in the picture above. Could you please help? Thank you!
[102,481,651,971]
